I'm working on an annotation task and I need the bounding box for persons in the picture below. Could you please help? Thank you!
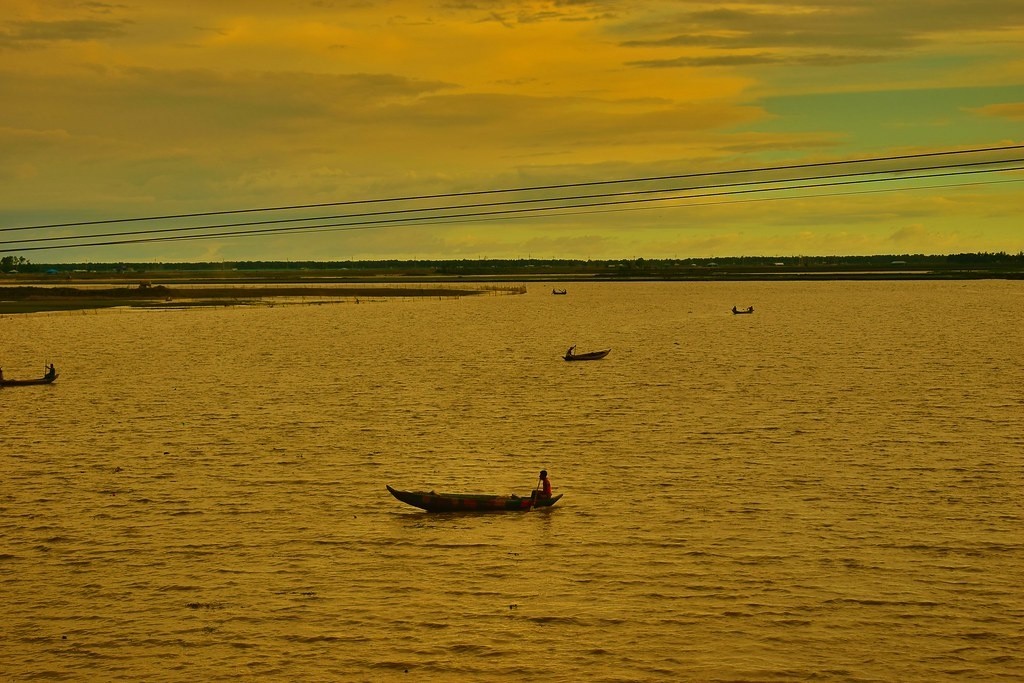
[566,345,575,356]
[46,364,55,377]
[532,470,551,498]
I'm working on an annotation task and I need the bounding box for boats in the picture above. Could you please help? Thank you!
[0,371,62,387]
[385,484,565,511]
[561,347,612,361]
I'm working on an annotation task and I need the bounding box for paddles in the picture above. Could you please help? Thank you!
[532,477,543,509]
[42,357,48,379]
[573,345,576,359]
